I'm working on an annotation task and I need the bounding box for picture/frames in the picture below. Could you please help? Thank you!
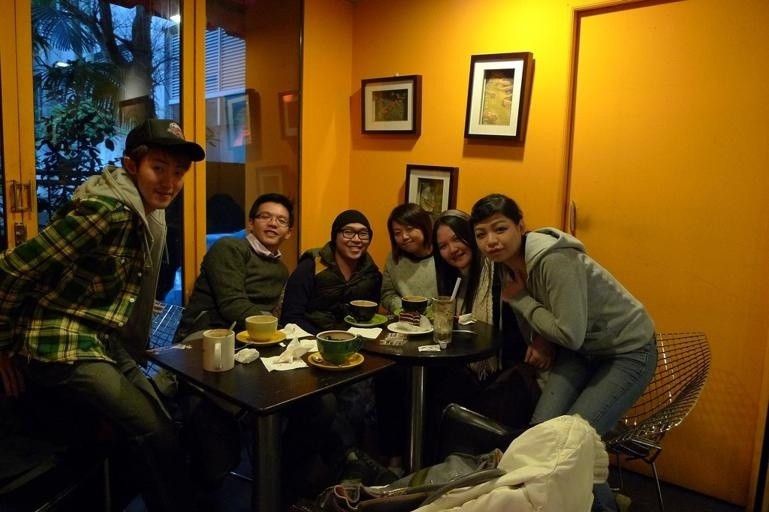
[363,74,420,135]
[404,164,458,212]
[277,91,299,141]
[116,94,158,134]
[464,51,533,143]
[224,89,257,149]
[256,166,286,195]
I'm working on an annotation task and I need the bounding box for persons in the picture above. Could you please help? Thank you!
[282,207,414,484]
[0,116,206,512]
[154,193,294,507]
[472,194,660,512]
[430,205,536,465]
[378,200,439,480]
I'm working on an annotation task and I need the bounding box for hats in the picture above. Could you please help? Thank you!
[124,118,205,162]
[331,210,372,242]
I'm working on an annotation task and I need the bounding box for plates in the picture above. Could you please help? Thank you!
[308,351,364,371]
[344,313,388,327]
[236,330,286,345]
[387,322,433,334]
[393,306,433,319]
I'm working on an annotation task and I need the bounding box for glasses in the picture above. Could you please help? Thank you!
[337,228,370,240]
[252,212,290,228]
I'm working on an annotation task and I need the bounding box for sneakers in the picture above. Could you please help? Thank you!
[387,462,411,478]
[344,447,391,480]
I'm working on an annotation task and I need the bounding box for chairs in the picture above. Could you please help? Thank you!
[137,299,256,484]
[590,331,711,509]
[1,385,112,511]
[287,399,623,512]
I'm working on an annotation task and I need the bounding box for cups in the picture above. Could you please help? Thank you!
[432,296,456,343]
[344,300,378,322]
[401,295,428,314]
[244,315,279,341]
[203,328,236,373]
[316,330,363,364]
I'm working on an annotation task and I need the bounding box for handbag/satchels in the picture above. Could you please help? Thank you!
[305,448,505,512]
